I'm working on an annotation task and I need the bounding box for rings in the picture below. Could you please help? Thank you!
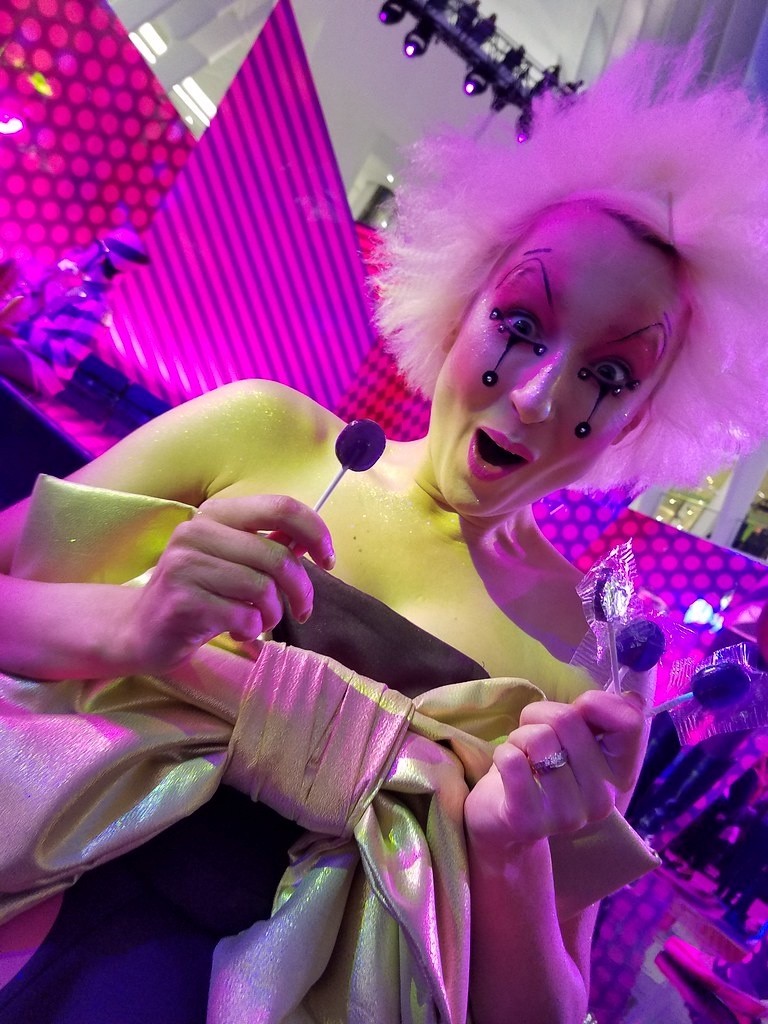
[532,748,569,772]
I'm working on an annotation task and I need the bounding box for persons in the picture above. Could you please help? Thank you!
[0,42,768,1024]
[631,726,768,938]
[0,228,152,397]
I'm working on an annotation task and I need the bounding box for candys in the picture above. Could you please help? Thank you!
[590,568,754,743]
[289,418,386,550]
